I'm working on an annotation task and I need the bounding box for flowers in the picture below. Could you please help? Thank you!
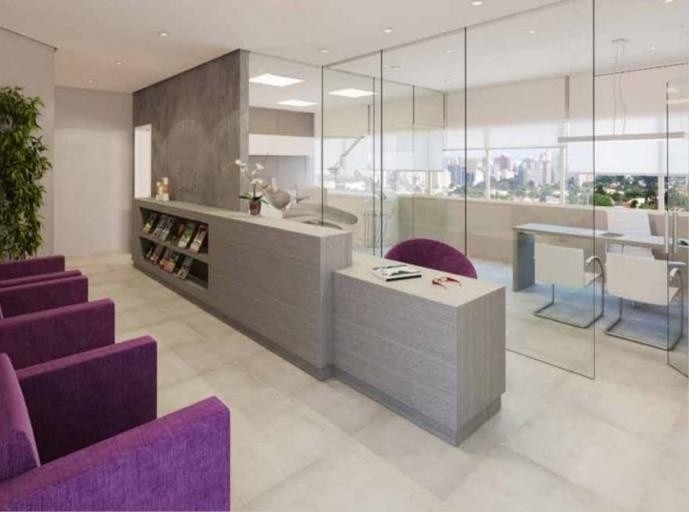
[234,159,279,204]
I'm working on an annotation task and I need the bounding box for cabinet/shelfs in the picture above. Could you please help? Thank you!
[138,205,208,291]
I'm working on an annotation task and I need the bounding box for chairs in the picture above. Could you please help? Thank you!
[533,205,684,351]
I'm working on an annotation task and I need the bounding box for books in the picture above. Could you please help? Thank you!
[142,213,208,280]
[371,264,423,281]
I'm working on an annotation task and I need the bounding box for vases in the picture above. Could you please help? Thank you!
[249,200,260,215]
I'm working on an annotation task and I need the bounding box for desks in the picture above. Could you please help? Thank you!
[513,223,689,293]
